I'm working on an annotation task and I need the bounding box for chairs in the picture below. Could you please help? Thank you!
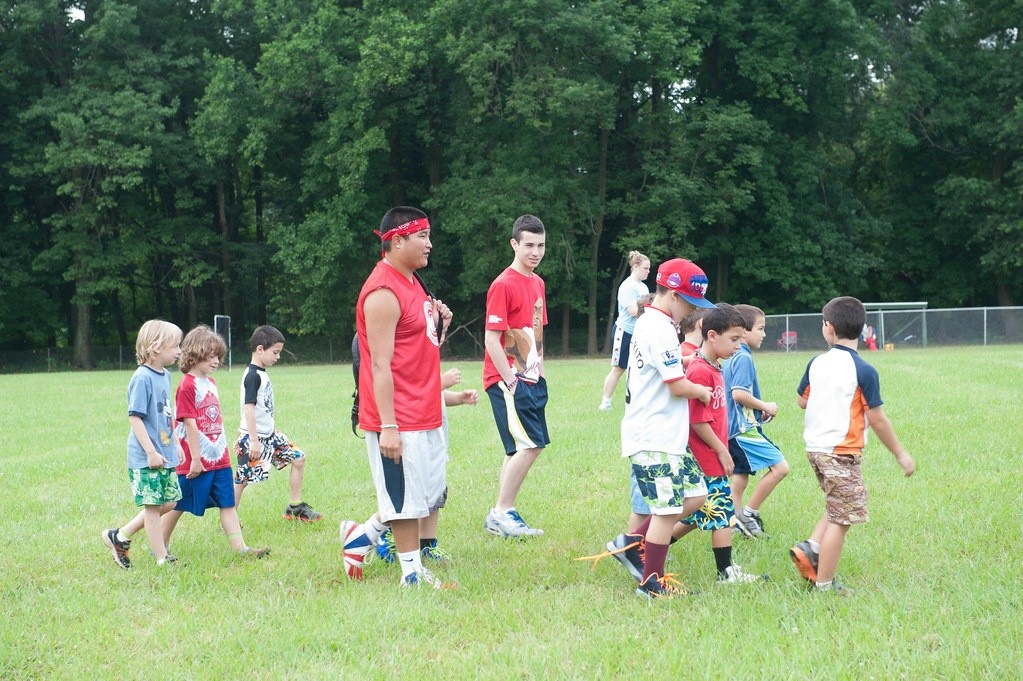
[776,330,798,352]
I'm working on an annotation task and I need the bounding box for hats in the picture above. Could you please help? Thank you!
[656,258,717,309]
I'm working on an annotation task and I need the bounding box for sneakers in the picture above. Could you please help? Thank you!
[790,540,819,585]
[735,507,771,543]
[572,532,646,583]
[102,528,133,570]
[485,507,544,542]
[283,502,322,522]
[636,572,701,601]
[400,567,460,592]
[155,555,189,570]
[716,560,770,584]
[340,520,378,583]
[422,539,453,562]
[812,578,855,598]
[376,527,398,565]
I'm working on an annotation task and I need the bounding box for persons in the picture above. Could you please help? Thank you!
[720,304,789,538]
[150,327,270,558]
[233,325,322,523]
[787,297,915,599]
[102,320,186,569]
[482,215,548,538]
[340,205,478,593]
[606,259,768,599]
[599,249,650,409]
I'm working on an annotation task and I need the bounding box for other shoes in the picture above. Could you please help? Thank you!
[598,403,613,410]
[150,548,178,563]
[245,548,270,560]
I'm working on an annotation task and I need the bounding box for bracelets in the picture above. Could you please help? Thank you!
[507,378,518,389]
[380,424,399,429]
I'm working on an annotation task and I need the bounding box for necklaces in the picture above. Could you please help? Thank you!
[695,349,723,371]
